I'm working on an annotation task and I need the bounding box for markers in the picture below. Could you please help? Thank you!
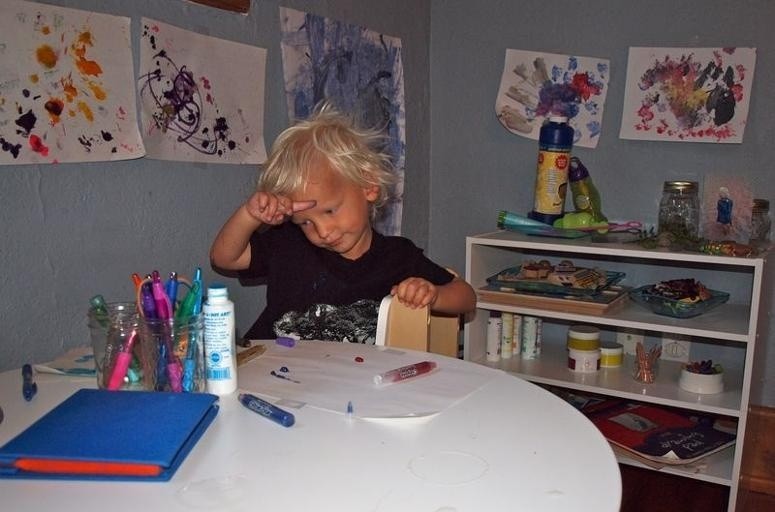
[374,362,439,386]
[275,337,294,348]
[237,393,295,427]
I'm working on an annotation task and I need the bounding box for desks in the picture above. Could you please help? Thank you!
[0,339,626,510]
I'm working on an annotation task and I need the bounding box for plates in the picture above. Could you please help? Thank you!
[627,282,732,321]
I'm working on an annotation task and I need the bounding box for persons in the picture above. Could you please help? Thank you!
[211,97,477,346]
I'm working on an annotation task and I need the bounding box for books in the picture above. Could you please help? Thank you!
[535,383,737,471]
[0,387,221,483]
[591,220,654,245]
[479,284,635,316]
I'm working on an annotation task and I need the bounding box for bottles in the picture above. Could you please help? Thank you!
[530,114,608,223]
[656,179,701,243]
[752,196,771,248]
[485,310,543,362]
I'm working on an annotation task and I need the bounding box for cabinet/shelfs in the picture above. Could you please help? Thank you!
[462,230,769,511]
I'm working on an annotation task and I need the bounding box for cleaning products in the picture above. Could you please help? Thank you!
[487,311,542,362]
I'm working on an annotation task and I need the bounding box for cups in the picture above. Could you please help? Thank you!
[85,300,206,394]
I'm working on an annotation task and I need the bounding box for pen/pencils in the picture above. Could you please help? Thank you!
[236,337,267,367]
[635,342,662,383]
[91,267,203,394]
[21,363,38,401]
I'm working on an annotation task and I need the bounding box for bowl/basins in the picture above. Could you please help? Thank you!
[679,362,725,394]
